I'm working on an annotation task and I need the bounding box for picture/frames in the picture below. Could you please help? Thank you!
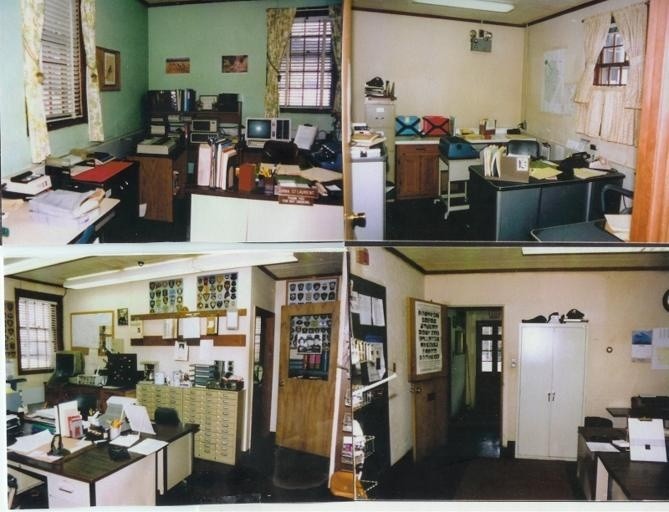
[94,44,121,93]
[282,275,341,352]
[454,329,466,355]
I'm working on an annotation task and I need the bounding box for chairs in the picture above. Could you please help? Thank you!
[262,139,299,165]
[505,137,541,162]
[70,393,102,418]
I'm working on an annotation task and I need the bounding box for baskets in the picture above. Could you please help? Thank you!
[351,385,372,412]
[356,479,377,499]
[353,436,376,464]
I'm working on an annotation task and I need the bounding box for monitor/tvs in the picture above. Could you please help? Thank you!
[244,117,276,142]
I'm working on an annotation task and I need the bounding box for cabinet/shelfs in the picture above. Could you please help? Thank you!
[45,156,141,243]
[0,182,122,246]
[396,136,441,202]
[350,381,379,501]
[513,317,589,465]
[125,143,189,225]
[182,169,347,243]
[135,374,247,469]
[463,155,632,244]
[139,98,243,142]
[350,146,388,241]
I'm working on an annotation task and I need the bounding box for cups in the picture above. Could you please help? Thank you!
[109,425,121,441]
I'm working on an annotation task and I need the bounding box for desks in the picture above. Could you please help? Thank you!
[572,405,669,501]
[8,399,202,510]
[428,131,543,223]
[42,381,135,414]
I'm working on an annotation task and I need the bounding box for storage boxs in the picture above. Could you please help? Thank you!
[363,94,399,205]
[440,169,460,192]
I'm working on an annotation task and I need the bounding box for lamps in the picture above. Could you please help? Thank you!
[470,29,493,52]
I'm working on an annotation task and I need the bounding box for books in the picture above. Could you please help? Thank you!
[54,400,84,438]
[352,131,385,147]
[483,143,507,178]
[196,136,237,191]
[31,186,106,234]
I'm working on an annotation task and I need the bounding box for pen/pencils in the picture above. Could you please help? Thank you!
[260,161,280,178]
[479,118,487,126]
[110,419,124,428]
